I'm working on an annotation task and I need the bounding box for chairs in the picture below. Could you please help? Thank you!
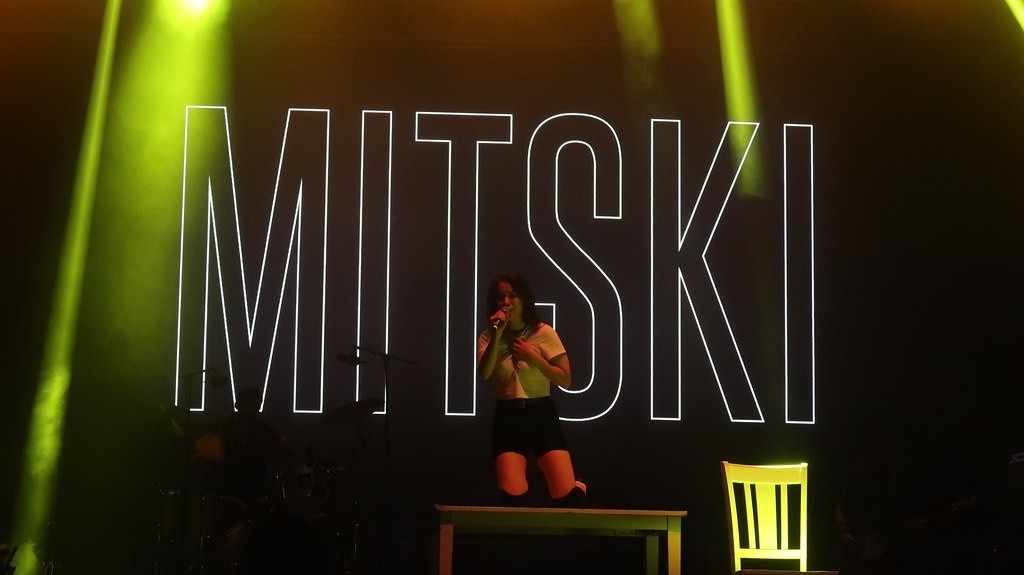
[719,460,840,575]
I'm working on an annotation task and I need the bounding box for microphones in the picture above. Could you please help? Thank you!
[337,353,367,364]
[208,375,230,388]
[492,307,510,331]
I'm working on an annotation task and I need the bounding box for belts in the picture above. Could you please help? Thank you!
[496,396,549,409]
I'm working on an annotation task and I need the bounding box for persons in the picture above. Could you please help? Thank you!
[476,273,588,507]
[834,436,928,575]
[212,390,293,575]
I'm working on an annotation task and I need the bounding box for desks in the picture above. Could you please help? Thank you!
[433,504,688,575]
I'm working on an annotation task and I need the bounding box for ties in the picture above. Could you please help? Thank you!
[502,320,545,374]
